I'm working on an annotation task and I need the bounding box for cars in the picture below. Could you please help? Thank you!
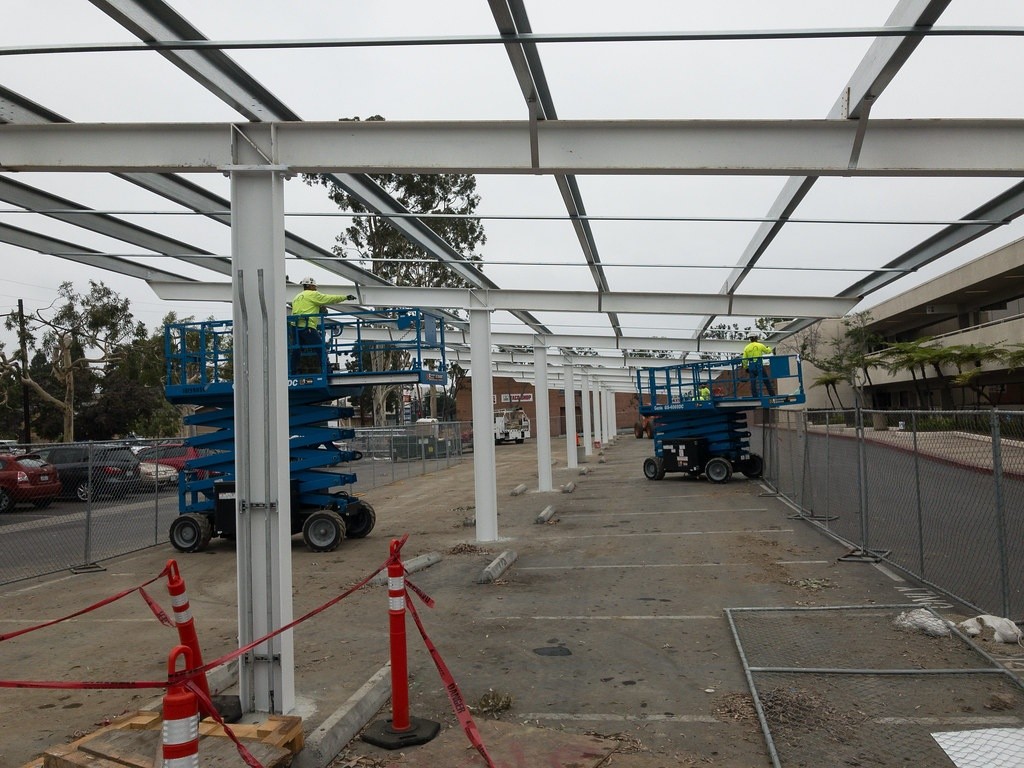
[138,460,179,493]
[0,453,63,514]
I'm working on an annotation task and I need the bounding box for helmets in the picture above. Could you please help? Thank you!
[300,277,318,286]
[748,332,757,337]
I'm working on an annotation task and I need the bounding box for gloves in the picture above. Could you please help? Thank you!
[746,368,749,373]
[347,295,357,300]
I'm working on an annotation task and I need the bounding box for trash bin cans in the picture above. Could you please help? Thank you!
[871,409,889,431]
[416,418,440,440]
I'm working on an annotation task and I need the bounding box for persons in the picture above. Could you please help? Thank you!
[742,331,775,398]
[691,384,710,401]
[291,276,357,373]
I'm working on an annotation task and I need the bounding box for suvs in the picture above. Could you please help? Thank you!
[26,445,144,504]
[131,443,206,482]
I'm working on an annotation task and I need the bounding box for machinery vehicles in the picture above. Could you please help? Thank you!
[634,402,664,439]
[163,306,446,552]
[636,353,807,485]
[493,407,531,444]
